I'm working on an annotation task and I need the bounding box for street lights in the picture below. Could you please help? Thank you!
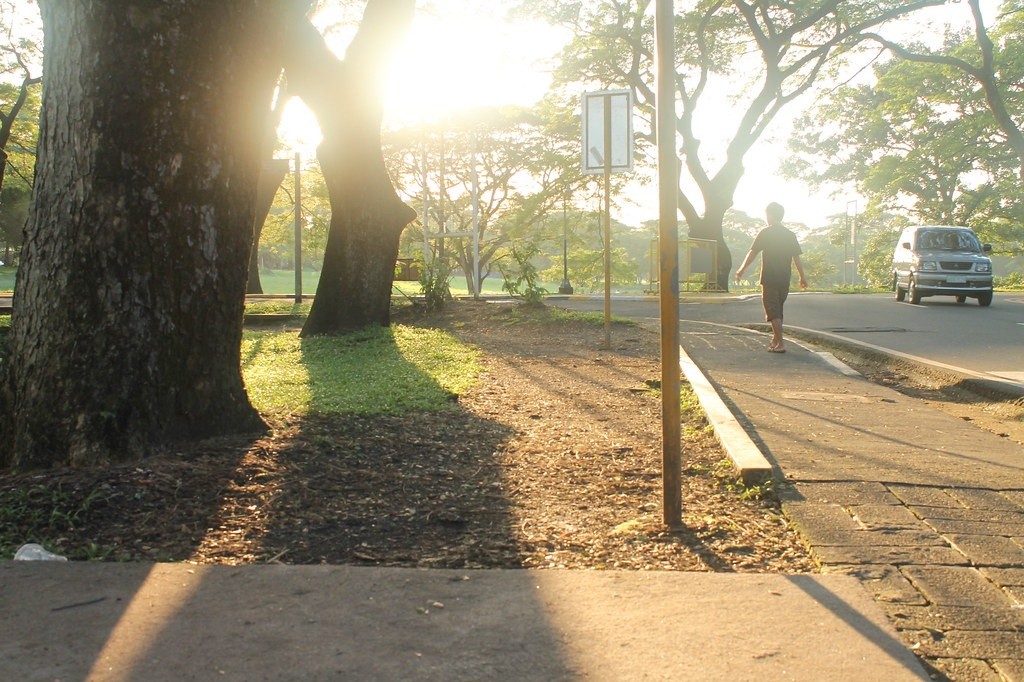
[560,183,574,294]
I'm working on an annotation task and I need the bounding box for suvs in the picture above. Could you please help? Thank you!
[890,224,994,306]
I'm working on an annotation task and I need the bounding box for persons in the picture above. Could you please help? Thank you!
[735,201,808,353]
